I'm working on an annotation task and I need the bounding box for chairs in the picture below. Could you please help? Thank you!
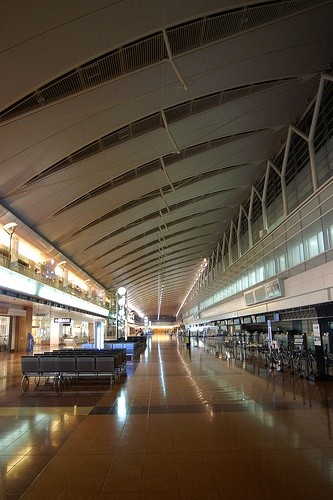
[16,348,128,397]
[81,334,146,357]
